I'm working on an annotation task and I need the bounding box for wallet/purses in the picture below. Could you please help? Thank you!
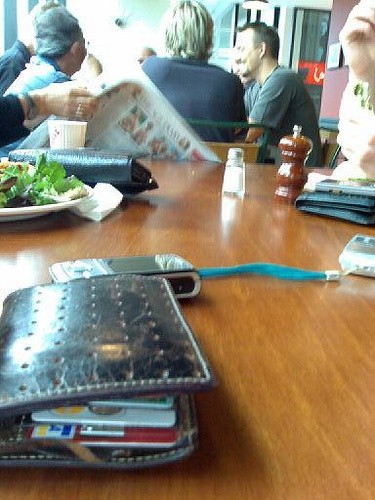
[0,276,212,469]
[294,192,375,224]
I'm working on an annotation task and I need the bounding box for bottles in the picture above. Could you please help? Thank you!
[221,147,248,199]
[275,125,312,203]
[8,149,160,196]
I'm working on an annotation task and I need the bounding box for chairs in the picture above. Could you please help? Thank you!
[316,115,346,171]
[186,118,271,162]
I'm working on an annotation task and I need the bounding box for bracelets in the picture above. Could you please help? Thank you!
[21,91,38,120]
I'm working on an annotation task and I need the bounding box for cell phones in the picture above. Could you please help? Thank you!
[47,253,200,297]
[339,233,375,277]
[314,179,375,196]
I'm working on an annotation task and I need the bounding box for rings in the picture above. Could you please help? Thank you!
[76,112,85,118]
[77,104,86,111]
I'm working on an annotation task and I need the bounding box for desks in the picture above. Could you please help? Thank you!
[0,160,375,500]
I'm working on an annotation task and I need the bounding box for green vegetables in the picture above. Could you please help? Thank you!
[0,152,84,208]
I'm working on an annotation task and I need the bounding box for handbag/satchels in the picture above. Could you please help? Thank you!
[9,148,157,192]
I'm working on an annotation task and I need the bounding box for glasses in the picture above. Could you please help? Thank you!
[73,40,89,49]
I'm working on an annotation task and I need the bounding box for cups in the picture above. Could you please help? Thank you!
[47,119,88,150]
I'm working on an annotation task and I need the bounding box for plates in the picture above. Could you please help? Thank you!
[0,180,95,223]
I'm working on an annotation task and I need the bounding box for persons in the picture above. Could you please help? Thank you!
[0,2,104,159]
[337,0,375,111]
[229,20,324,167]
[0,80,99,136]
[137,0,248,144]
